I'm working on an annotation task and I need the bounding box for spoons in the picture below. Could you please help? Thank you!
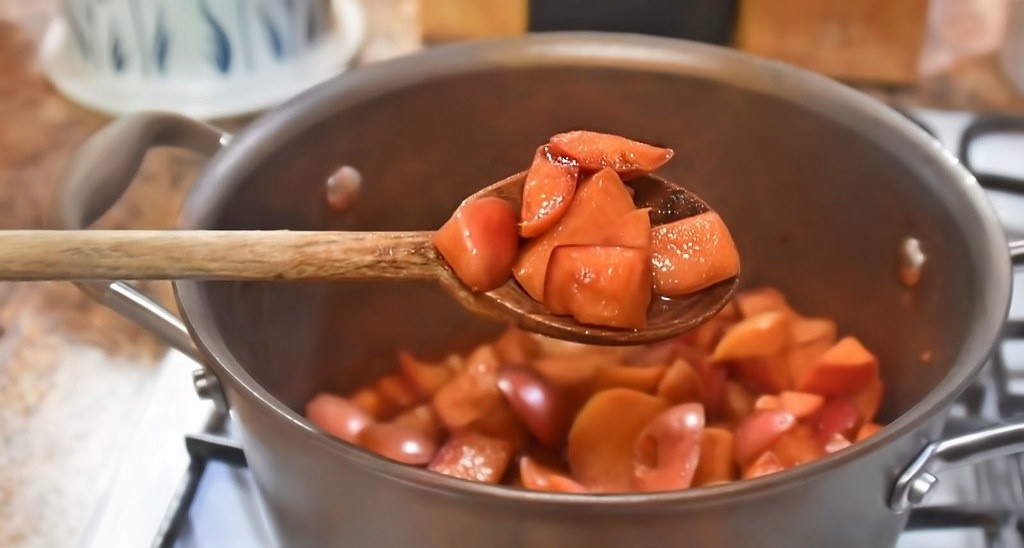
[0,166,741,346]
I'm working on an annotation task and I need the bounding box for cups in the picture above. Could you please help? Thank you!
[56,1,319,82]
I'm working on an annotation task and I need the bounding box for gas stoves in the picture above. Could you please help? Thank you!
[149,102,1024,548]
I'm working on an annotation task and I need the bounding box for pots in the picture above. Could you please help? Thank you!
[46,29,1024,548]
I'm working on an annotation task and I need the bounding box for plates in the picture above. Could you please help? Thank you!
[37,1,364,124]
